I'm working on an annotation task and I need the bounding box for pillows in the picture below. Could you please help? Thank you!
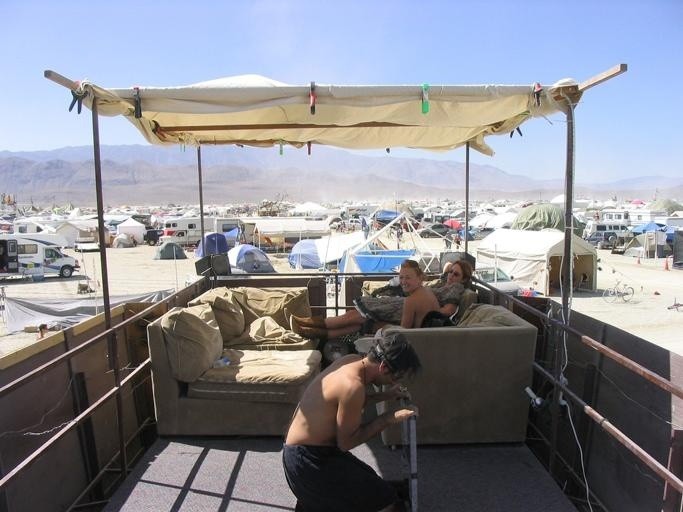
[163,289,245,384]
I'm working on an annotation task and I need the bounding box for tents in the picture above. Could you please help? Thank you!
[476,227,600,297]
[0,194,683,276]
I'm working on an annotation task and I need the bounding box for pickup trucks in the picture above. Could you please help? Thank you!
[340,219,363,231]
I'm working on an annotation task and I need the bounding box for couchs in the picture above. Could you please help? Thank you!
[144,286,325,448]
[362,277,535,449]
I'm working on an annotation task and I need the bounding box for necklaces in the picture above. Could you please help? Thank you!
[357,354,369,389]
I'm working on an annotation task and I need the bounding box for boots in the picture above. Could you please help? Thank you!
[283,308,327,340]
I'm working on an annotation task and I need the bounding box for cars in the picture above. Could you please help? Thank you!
[471,262,523,297]
[415,224,453,238]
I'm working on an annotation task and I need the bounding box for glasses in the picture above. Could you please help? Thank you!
[449,268,461,277]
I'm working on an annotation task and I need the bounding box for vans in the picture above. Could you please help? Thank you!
[0,235,80,279]
[581,207,668,250]
[158,218,215,248]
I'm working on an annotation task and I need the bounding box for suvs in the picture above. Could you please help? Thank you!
[375,209,417,232]
[143,230,164,246]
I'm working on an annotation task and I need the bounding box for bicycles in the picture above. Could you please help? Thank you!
[602,279,635,304]
[667,298,683,312]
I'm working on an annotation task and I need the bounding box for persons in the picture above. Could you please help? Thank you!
[280,331,423,511]
[282,258,474,342]
[373,258,441,341]
